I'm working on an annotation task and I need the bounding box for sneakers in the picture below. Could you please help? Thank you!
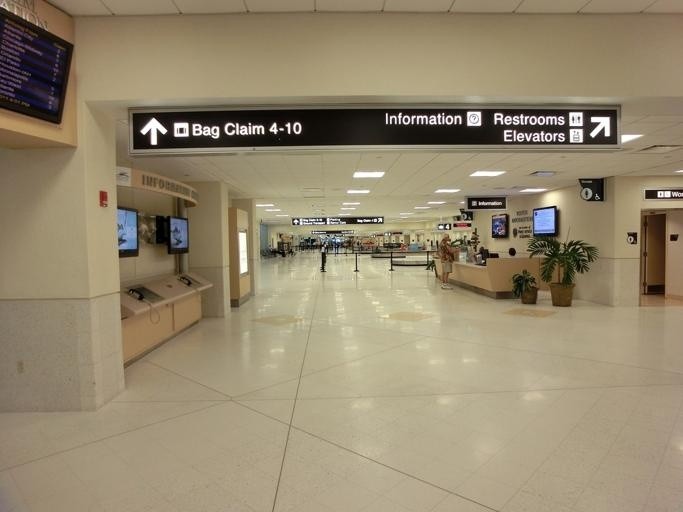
[441,283,453,289]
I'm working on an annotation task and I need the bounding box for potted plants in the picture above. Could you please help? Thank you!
[526,230,598,307]
[512,268,541,304]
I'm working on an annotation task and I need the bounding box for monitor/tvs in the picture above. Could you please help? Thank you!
[0,6,75,124]
[533,206,558,236]
[167,216,189,255]
[117,206,139,258]
[491,214,508,238]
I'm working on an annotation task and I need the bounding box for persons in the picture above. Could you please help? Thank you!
[400,238,432,252]
[323,234,339,253]
[507,248,518,257]
[439,234,464,290]
